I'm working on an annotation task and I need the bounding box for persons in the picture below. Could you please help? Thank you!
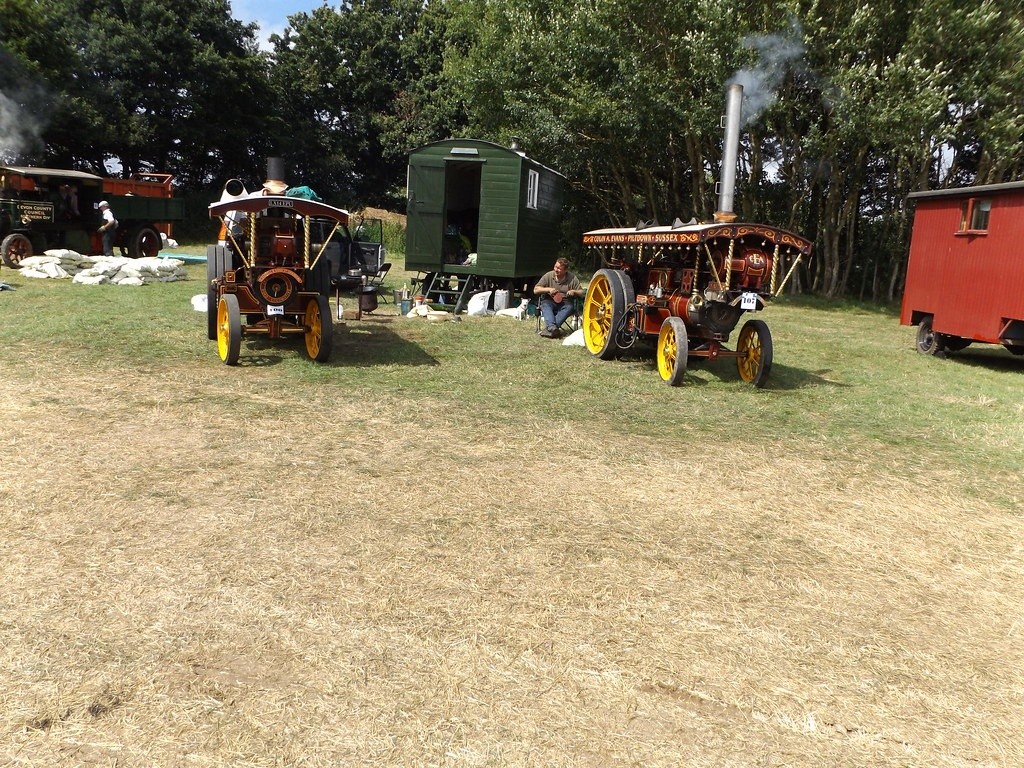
[533,258,584,339]
[97,200,119,255]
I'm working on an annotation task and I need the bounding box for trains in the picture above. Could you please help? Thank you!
[204,156,350,369]
[897,181,1024,360]
[576,80,812,389]
[0,171,176,241]
[0,164,184,270]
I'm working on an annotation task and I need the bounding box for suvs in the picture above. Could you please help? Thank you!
[213,204,385,295]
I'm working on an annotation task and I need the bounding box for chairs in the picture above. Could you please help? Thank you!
[359,260,391,303]
[537,294,580,333]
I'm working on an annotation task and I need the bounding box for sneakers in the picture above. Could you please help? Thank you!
[550,326,558,332]
[540,329,553,337]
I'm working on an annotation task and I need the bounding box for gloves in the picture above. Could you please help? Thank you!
[97,225,106,234]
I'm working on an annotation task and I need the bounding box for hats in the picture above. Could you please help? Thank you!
[98,201,109,207]
